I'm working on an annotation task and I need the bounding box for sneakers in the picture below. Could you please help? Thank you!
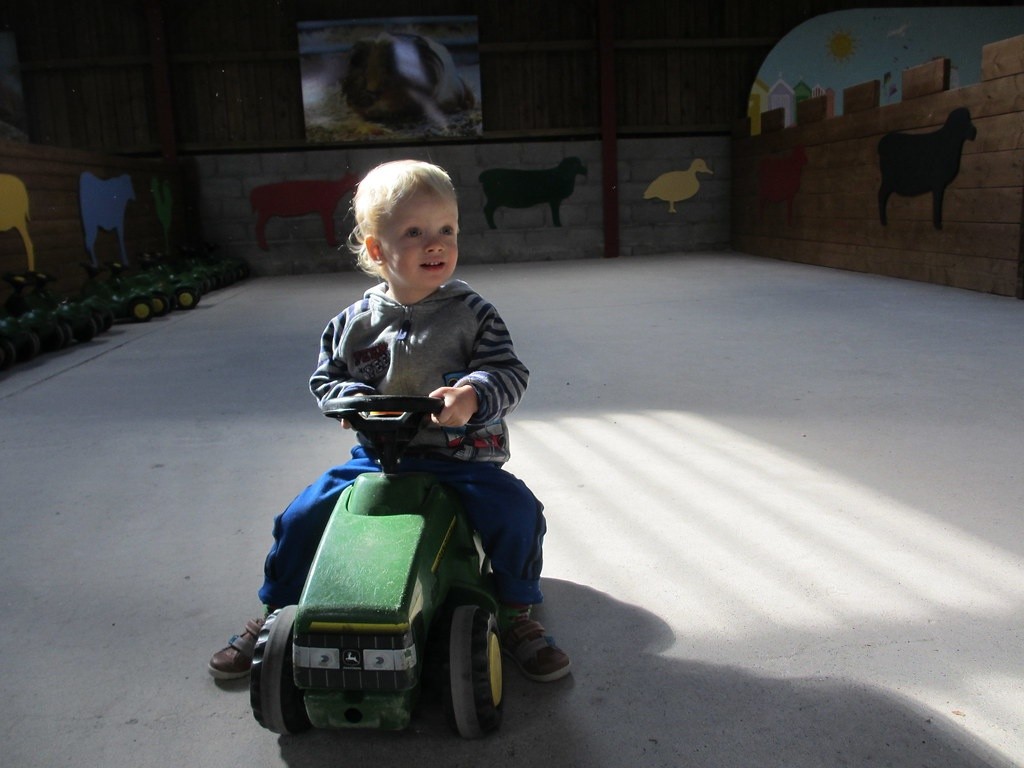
[205,614,268,679]
[500,619,569,682]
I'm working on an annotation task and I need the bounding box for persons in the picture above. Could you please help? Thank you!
[208,160,573,682]
